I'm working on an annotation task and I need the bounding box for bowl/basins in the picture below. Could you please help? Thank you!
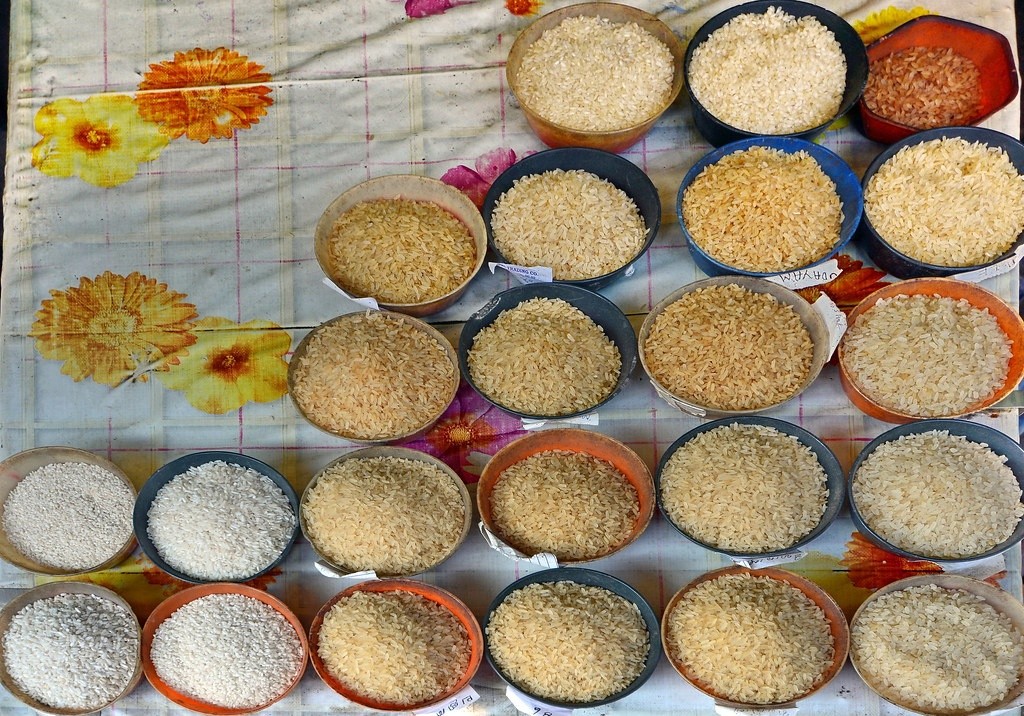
[1,417,1024,715]
[285,125,1023,444]
[505,0,1019,147]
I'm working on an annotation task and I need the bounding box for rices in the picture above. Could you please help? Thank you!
[0,6,1024,716]
